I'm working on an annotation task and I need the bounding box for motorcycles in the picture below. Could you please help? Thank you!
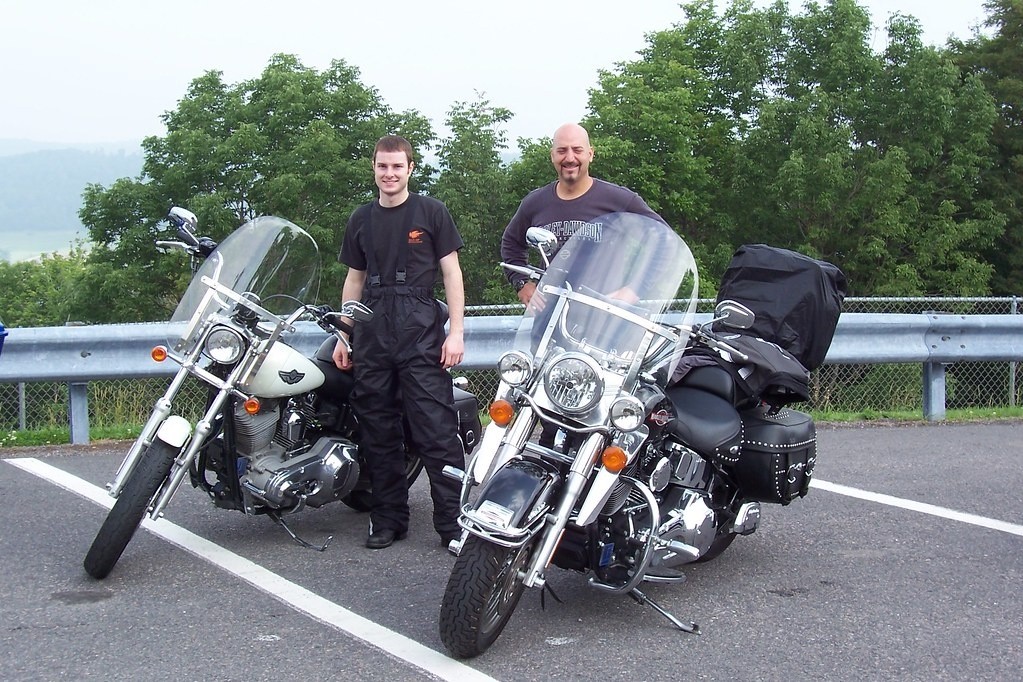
[83,208,484,577]
[439,210,850,658]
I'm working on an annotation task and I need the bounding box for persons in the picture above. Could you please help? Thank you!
[500,123,669,472]
[332,134,466,556]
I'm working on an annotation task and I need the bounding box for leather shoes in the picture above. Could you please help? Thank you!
[440,532,462,548]
[366,529,407,548]
